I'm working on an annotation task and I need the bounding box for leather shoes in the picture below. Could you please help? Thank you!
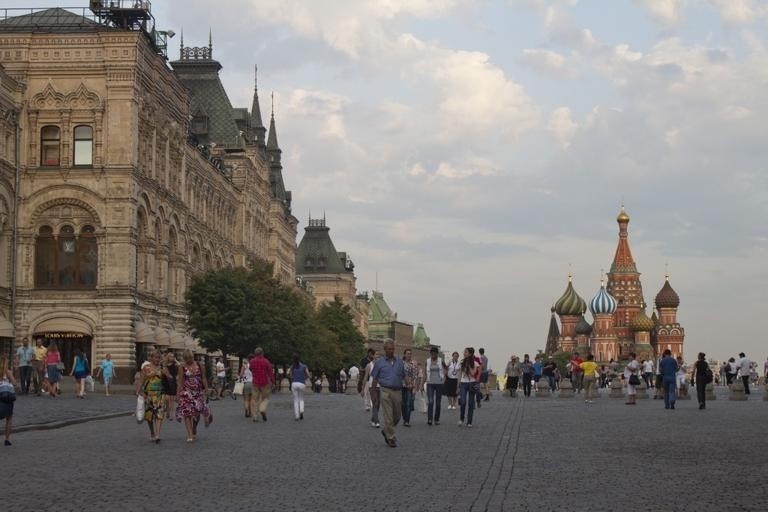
[383,431,397,448]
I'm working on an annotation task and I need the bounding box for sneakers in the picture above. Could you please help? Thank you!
[371,421,379,428]
[4,440,12,446]
[585,399,594,403]
[699,403,705,409]
[448,405,456,410]
[434,421,440,425]
[186,438,196,443]
[150,435,160,442]
[404,422,410,426]
[295,418,299,421]
[252,418,258,422]
[458,421,463,427]
[625,402,636,405]
[665,404,674,409]
[300,412,303,419]
[260,411,267,421]
[428,420,432,425]
[467,423,472,427]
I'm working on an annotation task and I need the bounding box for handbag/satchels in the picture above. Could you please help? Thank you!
[629,367,641,385]
[135,394,145,424]
[85,376,94,392]
[232,378,244,395]
[203,396,213,428]
[724,364,731,372]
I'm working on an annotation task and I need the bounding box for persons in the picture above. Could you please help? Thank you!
[15,337,118,399]
[239,347,311,422]
[136,349,209,443]
[657,349,710,410]
[359,338,418,446]
[215,356,230,400]
[417,346,488,428]
[504,354,561,397]
[1,353,17,445]
[311,363,360,393]
[713,351,759,394]
[568,352,654,405]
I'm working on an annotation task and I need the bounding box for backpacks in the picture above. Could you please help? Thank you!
[704,363,713,384]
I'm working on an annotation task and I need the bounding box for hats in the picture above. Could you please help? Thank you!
[430,348,438,353]
